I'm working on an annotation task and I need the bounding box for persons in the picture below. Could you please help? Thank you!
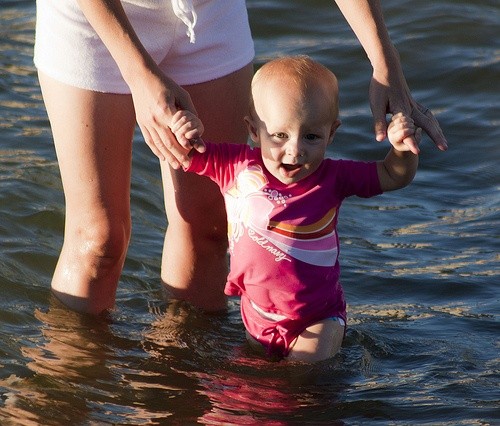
[168,54,419,364]
[32,0,450,316]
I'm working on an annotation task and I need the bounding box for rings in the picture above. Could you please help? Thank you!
[422,106,428,113]
